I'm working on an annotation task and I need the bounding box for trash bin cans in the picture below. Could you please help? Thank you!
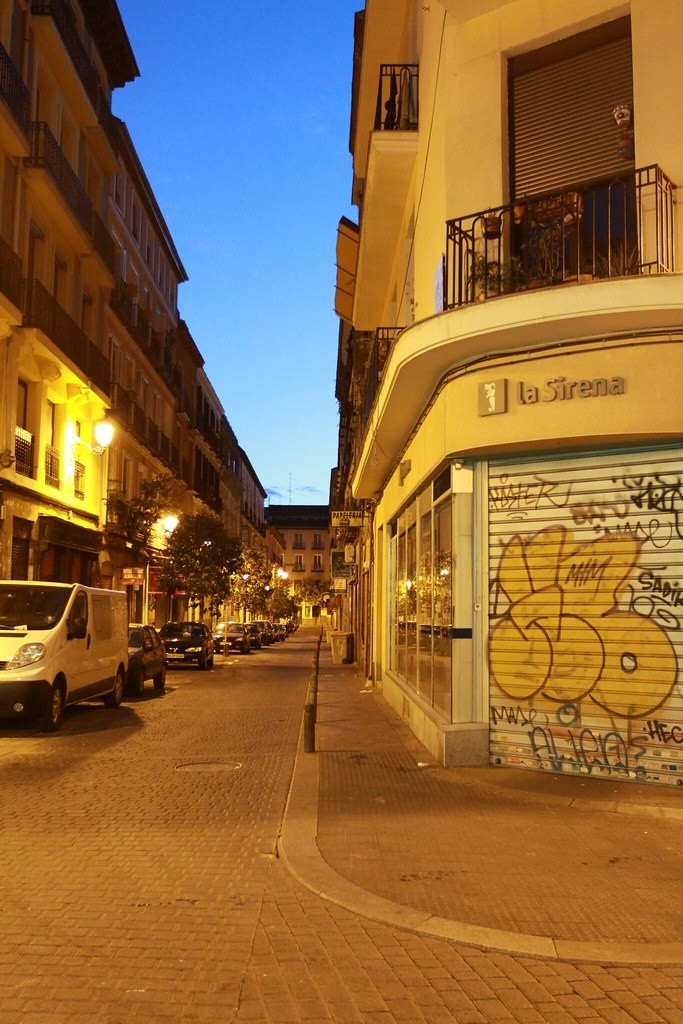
[324,627,338,645]
[330,631,353,665]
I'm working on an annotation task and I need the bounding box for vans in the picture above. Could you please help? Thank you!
[0,580,129,732]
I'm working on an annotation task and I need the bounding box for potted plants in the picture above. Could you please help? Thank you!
[479,206,502,240]
[464,253,515,303]
[565,261,599,283]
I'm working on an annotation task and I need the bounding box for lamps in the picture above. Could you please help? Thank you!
[70,421,113,456]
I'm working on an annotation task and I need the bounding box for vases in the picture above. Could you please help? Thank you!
[612,105,631,129]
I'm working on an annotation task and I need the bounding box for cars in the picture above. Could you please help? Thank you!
[212,621,251,654]
[158,621,214,669]
[243,623,262,649]
[254,620,287,645]
[128,623,167,696]
